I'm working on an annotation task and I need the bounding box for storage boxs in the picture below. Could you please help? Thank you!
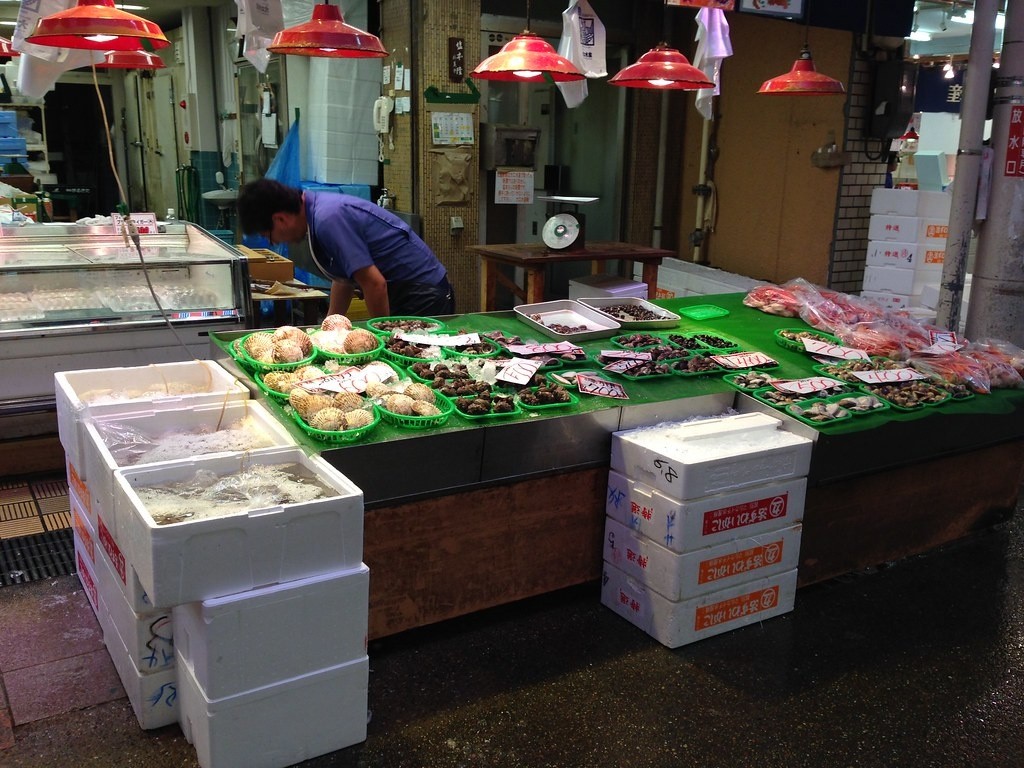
[54,359,371,768]
[308,55,380,187]
[601,411,814,646]
[860,188,971,322]
[568,275,649,303]
[0,111,27,163]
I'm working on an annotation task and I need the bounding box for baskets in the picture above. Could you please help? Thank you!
[228,316,502,443]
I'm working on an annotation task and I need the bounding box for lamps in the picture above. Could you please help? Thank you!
[606,0,717,89]
[24,0,172,51]
[266,0,389,58]
[469,0,586,82]
[756,0,847,94]
[0,36,20,56]
[92,50,167,69]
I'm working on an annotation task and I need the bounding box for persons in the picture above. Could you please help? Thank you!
[237,178,455,317]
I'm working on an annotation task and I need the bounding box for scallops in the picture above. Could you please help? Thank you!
[244,314,442,431]
[733,369,883,424]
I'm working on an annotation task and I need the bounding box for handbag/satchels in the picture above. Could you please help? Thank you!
[264,120,300,193]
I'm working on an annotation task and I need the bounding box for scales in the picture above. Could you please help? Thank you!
[535,195,600,251]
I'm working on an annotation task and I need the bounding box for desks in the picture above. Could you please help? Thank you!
[465,241,678,312]
[252,279,329,325]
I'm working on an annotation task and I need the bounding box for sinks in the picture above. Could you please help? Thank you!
[201,189,241,210]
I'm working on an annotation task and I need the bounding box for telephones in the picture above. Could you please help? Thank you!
[373,96,394,134]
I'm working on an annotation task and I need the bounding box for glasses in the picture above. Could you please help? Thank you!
[267,222,276,247]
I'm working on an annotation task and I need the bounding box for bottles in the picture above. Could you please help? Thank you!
[166,209,176,225]
